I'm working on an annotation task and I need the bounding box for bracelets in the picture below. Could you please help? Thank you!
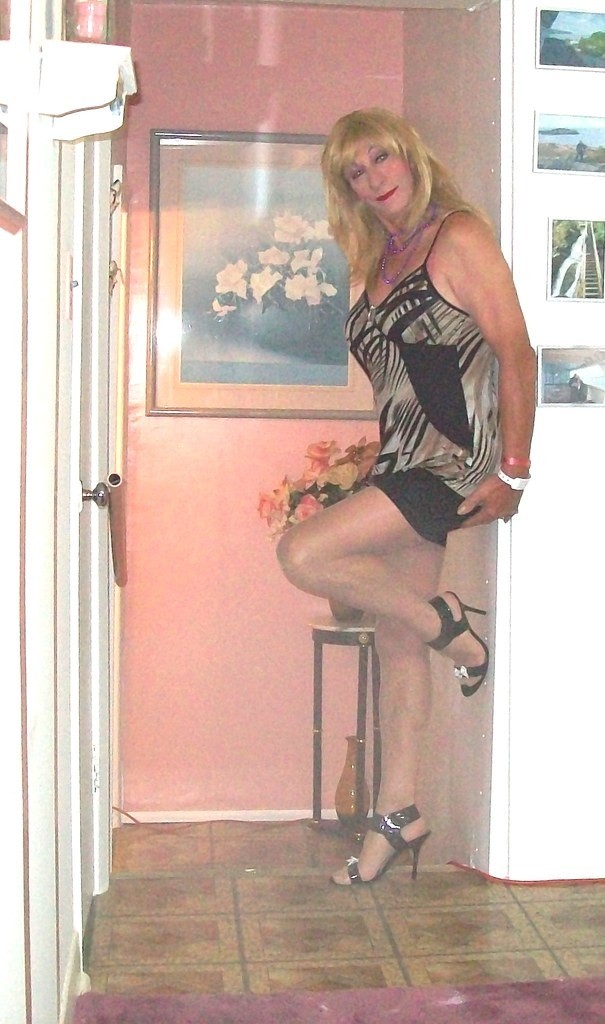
[498,468,532,491]
[505,456,532,468]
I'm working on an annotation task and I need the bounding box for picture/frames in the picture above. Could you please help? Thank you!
[547,217,605,302]
[145,127,374,422]
[536,7,605,71]
[533,110,605,175]
[536,346,605,407]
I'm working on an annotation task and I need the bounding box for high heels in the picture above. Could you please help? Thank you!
[331,804,431,885]
[422,589,490,696]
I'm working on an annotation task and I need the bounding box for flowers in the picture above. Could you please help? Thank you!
[259,434,385,541]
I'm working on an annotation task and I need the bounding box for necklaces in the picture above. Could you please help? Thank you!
[377,190,437,284]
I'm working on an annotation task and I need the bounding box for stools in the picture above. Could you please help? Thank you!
[308,615,388,842]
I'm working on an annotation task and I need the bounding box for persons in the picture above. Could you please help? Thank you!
[277,108,541,886]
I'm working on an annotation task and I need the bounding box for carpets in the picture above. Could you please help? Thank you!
[74,976,605,1024]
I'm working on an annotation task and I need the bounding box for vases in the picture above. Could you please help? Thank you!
[336,735,370,822]
[329,599,364,621]
